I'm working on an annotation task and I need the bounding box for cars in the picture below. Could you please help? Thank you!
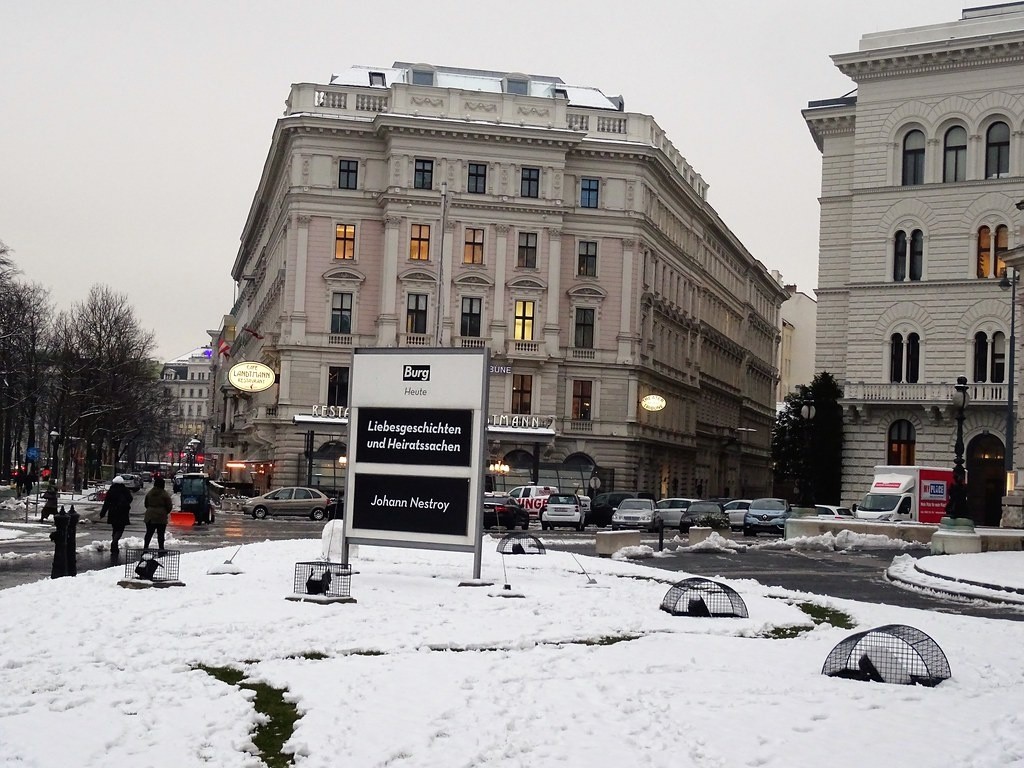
[815,503,855,521]
[655,497,704,527]
[611,497,663,536]
[120,471,153,492]
[679,501,729,536]
[480,491,530,530]
[539,492,586,531]
[743,497,795,538]
[243,486,333,521]
[722,499,753,527]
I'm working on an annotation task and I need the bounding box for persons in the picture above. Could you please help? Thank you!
[12,469,172,554]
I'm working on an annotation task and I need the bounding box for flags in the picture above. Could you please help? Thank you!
[242,327,263,339]
[218,339,230,354]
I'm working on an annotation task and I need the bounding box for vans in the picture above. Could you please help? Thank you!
[506,485,560,520]
[560,491,656,529]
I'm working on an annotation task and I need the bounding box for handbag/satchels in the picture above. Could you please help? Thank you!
[44,492,53,499]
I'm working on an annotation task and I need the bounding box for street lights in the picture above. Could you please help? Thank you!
[949,372,970,522]
[49,427,59,484]
[799,388,820,508]
[999,264,1018,497]
[185,434,201,473]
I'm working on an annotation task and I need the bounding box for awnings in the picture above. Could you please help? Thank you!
[293,414,556,444]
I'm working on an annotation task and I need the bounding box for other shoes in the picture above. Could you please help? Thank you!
[39,519,43,523]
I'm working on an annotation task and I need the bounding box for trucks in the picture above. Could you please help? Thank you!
[855,464,968,524]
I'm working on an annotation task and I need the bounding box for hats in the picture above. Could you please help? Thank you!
[111,476,125,483]
